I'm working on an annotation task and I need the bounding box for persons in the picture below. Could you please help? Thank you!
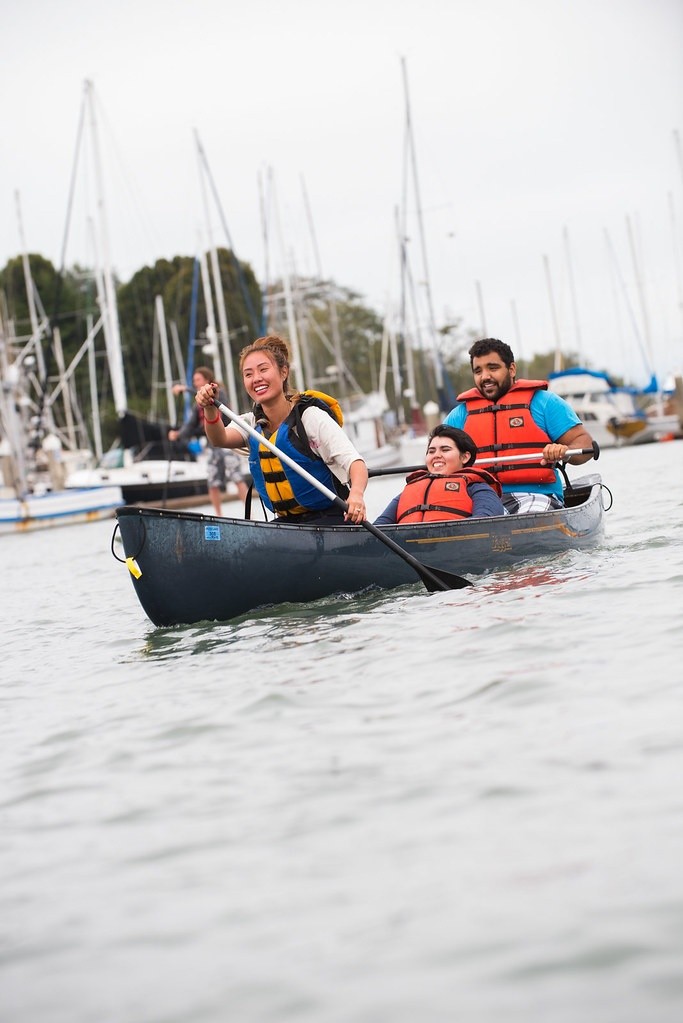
[194,336,369,526]
[442,337,593,514]
[168,366,250,516]
[373,424,504,525]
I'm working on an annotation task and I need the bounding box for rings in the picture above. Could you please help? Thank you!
[355,509,360,512]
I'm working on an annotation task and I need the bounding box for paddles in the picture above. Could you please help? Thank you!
[209,387,473,594]
[366,438,602,481]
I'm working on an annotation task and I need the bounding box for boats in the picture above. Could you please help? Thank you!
[114,473,603,628]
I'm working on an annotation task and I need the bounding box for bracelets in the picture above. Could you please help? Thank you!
[203,414,220,424]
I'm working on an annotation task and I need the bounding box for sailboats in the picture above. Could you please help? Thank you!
[0,55,683,535]
